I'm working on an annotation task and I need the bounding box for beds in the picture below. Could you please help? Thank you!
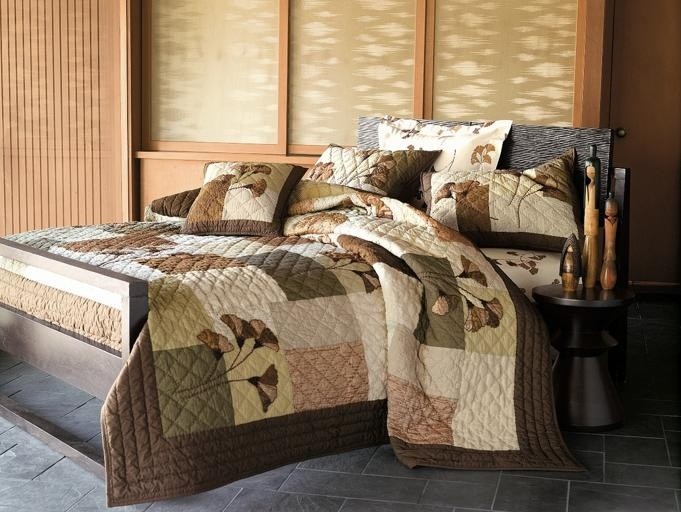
[0,104,644,507]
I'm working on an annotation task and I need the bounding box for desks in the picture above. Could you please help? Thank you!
[527,282,639,433]
